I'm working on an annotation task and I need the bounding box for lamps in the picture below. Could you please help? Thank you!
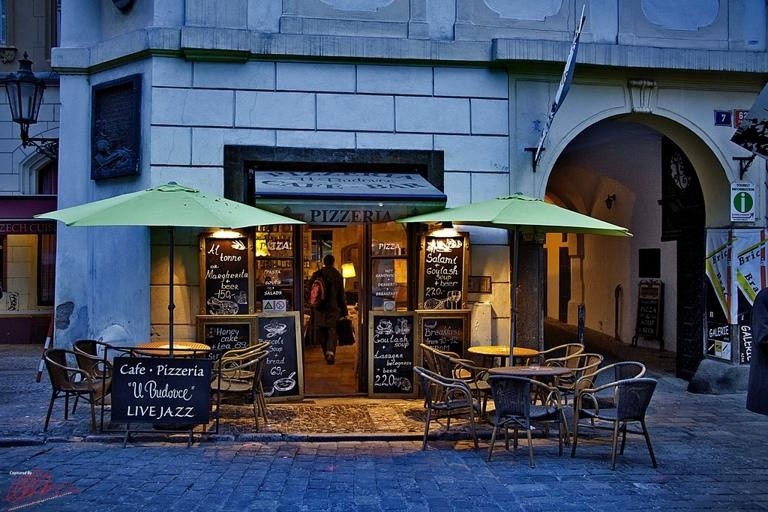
[439,220,453,228]
[605,190,616,212]
[2,50,59,160]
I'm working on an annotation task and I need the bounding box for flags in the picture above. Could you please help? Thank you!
[535,16,587,165]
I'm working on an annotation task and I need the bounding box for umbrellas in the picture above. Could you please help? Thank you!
[33,181,308,357]
[393,190,634,366]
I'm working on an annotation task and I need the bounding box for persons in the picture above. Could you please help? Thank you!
[307,254,348,364]
[746,287,768,416]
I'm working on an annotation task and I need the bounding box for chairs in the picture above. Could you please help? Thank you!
[411,340,661,472]
[39,336,268,436]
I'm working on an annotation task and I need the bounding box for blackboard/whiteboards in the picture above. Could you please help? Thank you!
[368,310,419,399]
[418,230,469,310]
[199,230,256,315]
[195,313,259,371]
[417,309,472,399]
[258,310,305,404]
[112,359,211,425]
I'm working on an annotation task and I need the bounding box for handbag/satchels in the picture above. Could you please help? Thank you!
[336,319,357,346]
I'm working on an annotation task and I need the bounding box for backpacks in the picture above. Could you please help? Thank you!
[306,269,327,310]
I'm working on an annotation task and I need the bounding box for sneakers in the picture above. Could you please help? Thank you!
[325,353,335,365]
